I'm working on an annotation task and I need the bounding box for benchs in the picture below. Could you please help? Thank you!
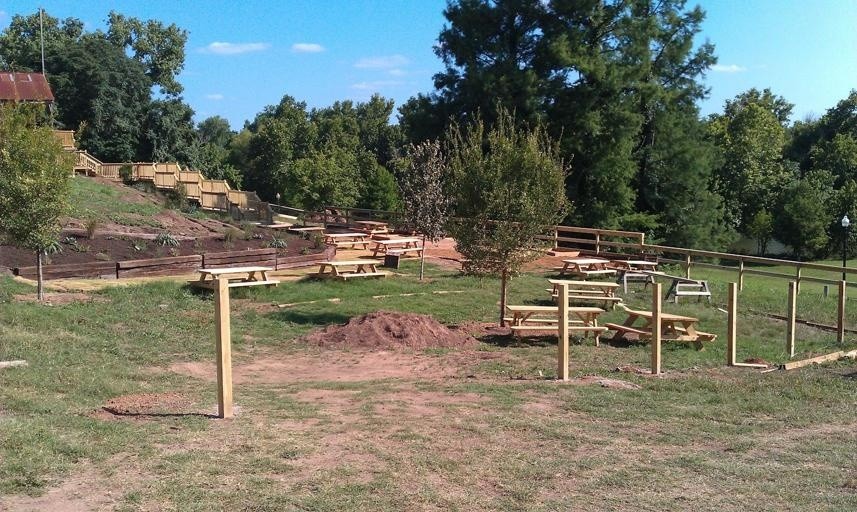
[458,259,503,272]
[503,258,717,353]
[187,221,427,298]
